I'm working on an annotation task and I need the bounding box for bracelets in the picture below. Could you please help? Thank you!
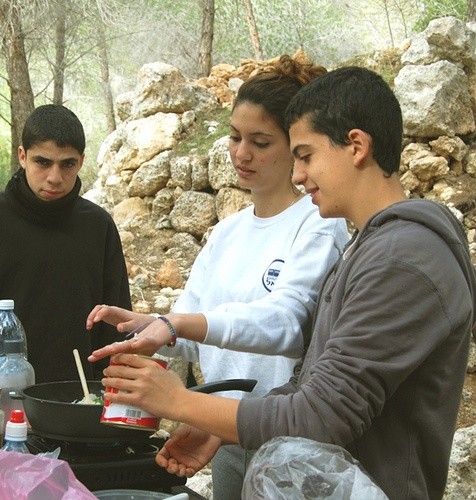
[158,316,178,348]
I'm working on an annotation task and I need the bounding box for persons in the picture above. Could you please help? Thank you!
[0,105,134,387]
[101,67,476,499]
[83,51,353,500]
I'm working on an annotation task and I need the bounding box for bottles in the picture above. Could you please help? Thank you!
[2,410,30,454]
[8,392,25,421]
[0,300,35,431]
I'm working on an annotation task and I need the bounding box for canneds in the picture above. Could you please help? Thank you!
[99,354,169,431]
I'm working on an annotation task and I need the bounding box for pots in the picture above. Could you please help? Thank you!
[8,379,257,442]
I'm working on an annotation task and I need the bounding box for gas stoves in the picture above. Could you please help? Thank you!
[24,427,187,489]
